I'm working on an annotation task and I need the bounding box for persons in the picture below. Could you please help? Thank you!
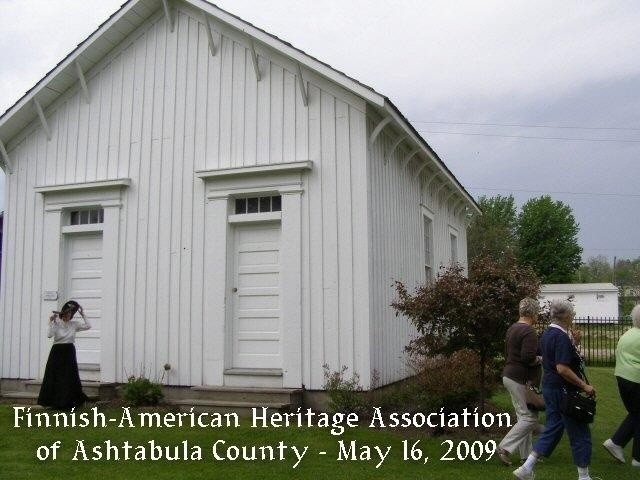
[495,297,544,466]
[36,300,92,409]
[601,304,640,467]
[512,298,597,480]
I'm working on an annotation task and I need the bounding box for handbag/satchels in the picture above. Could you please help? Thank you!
[525,379,546,412]
[558,384,597,424]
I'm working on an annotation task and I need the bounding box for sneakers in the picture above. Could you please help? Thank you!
[513,465,536,480]
[521,457,544,464]
[495,445,512,466]
[603,438,626,463]
[631,459,640,467]
